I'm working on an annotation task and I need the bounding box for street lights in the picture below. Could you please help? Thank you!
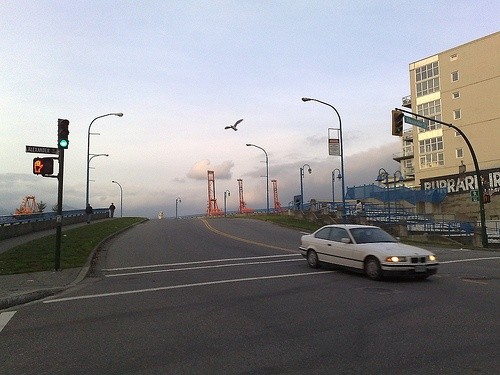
[246,143,269,216]
[332,169,342,211]
[176,197,181,218]
[224,190,230,215]
[302,98,346,222]
[86,112,124,222]
[112,181,122,218]
[393,170,405,221]
[375,168,390,222]
[300,164,312,209]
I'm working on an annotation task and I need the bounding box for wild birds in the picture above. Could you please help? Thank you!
[225,119,243,131]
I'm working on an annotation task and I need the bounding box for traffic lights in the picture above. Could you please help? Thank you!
[33,158,54,174]
[391,110,404,136]
[57,118,70,149]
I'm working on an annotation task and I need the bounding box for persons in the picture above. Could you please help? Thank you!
[86,204,93,223]
[356,200,363,209]
[109,203,115,218]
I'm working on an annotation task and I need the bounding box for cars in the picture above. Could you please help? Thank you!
[298,224,438,280]
